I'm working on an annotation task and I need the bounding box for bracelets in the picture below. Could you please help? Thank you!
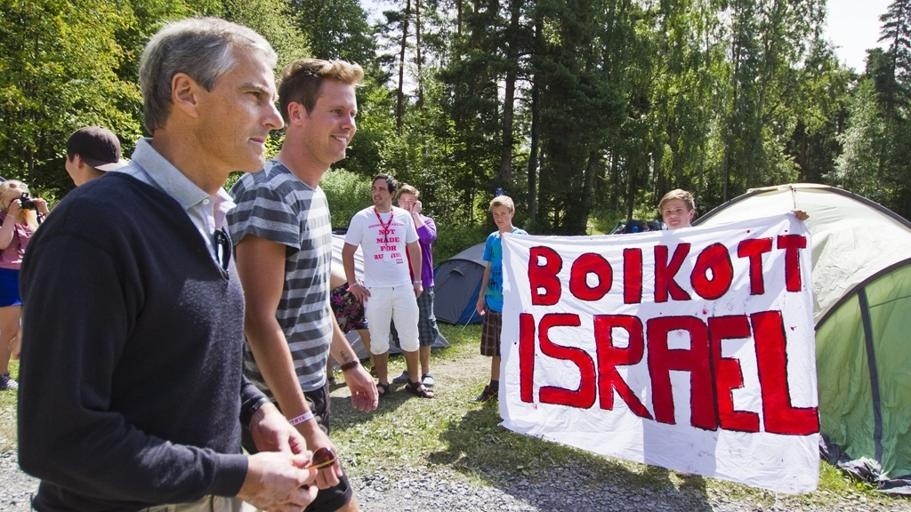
[6,214,16,218]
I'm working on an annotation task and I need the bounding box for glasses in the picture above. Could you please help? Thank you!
[304,445,336,471]
[214,226,231,271]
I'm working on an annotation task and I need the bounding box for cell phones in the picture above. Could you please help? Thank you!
[412,202,418,207]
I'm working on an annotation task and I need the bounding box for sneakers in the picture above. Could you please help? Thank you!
[422,373,434,387]
[327,376,337,387]
[0,372,19,391]
[393,371,409,383]
[369,365,378,378]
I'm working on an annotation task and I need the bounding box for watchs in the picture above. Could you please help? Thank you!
[341,359,359,371]
[246,397,271,426]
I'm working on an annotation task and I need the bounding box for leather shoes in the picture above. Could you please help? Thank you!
[468,385,498,404]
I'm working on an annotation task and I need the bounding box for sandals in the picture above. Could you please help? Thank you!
[405,379,434,398]
[376,382,389,398]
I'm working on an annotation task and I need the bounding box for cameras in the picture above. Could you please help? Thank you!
[10,192,35,210]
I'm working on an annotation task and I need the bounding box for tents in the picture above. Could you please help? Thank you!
[434,241,486,323]
[327,233,451,373]
[814,257,911,496]
[692,182,907,329]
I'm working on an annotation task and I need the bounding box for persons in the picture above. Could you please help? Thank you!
[66,126,119,186]
[393,184,438,391]
[0,178,50,391]
[658,188,810,231]
[327,274,376,390]
[476,195,529,402]
[342,174,435,398]
[227,60,378,512]
[17,16,318,512]
